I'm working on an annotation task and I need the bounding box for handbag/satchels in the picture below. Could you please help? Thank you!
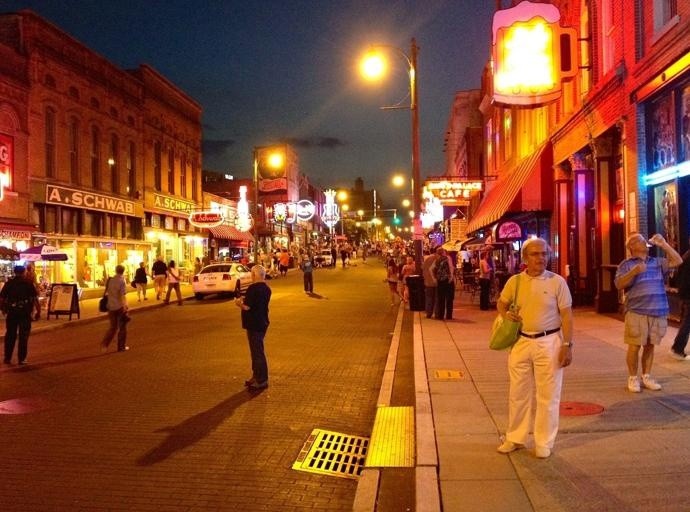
[489,304,520,350]
[100,295,107,312]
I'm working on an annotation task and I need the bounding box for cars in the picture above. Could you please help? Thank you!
[192,263,253,299]
[314,248,332,266]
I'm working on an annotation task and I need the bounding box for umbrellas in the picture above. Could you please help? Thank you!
[19,244,68,273]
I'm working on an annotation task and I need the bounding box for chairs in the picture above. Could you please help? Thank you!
[454,270,504,305]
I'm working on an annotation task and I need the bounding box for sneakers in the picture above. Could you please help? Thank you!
[498,441,524,453]
[118,347,129,352]
[669,348,690,360]
[19,360,28,365]
[536,446,551,458]
[628,373,661,393]
[245,378,268,389]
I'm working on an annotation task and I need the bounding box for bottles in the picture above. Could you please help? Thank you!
[646,233,663,247]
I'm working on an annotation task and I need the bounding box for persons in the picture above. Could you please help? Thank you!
[26,265,40,319]
[0,265,41,366]
[151,255,167,300]
[134,262,151,302]
[165,260,183,306]
[202,251,212,268]
[668,249,690,361]
[614,232,683,394]
[193,257,202,275]
[236,265,271,390]
[496,239,573,458]
[99,265,130,353]
[234,237,493,320]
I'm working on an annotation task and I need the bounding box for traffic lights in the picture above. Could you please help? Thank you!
[392,218,400,225]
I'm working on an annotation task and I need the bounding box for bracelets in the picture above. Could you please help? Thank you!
[561,342,573,347]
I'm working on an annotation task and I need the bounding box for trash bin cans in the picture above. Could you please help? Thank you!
[406,274,426,312]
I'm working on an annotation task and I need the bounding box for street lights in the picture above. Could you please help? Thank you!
[358,36,424,275]
[252,146,285,264]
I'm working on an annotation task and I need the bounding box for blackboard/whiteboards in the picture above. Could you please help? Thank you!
[49,284,76,315]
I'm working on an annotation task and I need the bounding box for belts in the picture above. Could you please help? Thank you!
[519,328,560,338]
